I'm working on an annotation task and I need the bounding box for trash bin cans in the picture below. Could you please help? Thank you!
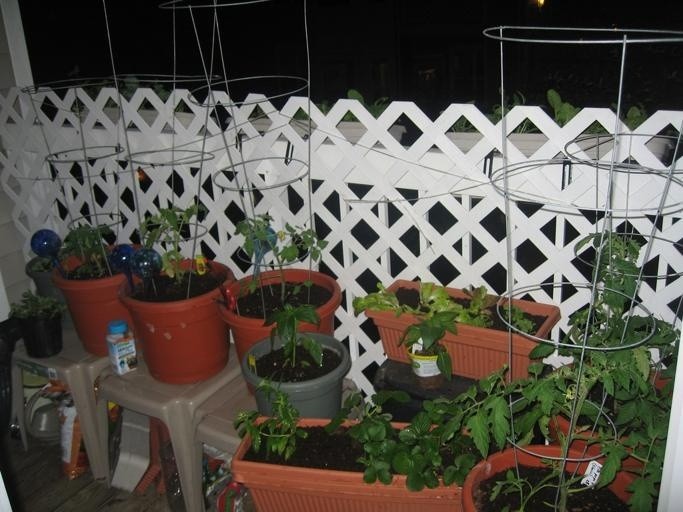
[158,442,185,511]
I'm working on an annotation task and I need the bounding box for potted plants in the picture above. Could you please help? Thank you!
[8,216,342,388]
[231,234,665,509]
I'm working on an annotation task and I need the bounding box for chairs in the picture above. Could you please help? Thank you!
[96,342,254,511]
[11,326,145,488]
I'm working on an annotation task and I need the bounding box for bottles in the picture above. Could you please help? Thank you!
[105,319,139,377]
